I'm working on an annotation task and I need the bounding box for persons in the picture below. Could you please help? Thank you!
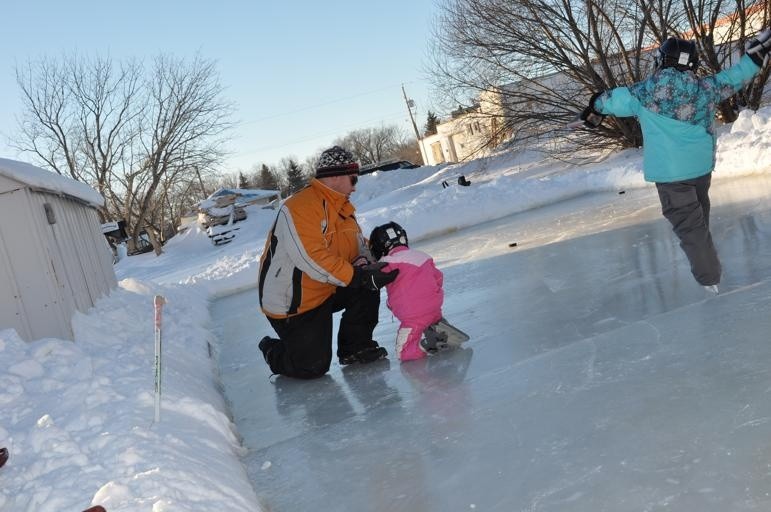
[369,221,471,362]
[259,146,401,378]
[591,28,771,294]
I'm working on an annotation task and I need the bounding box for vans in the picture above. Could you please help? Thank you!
[360,160,421,174]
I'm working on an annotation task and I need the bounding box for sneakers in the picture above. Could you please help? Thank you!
[338,342,389,367]
[421,319,472,354]
[258,336,282,376]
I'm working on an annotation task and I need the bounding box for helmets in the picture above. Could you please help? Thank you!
[368,220,411,258]
[650,37,702,75]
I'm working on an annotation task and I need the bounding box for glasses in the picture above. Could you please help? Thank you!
[342,174,361,188]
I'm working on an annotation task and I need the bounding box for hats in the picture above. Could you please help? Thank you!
[313,146,362,179]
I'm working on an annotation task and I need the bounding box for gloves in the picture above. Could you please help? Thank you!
[745,25,771,68]
[581,90,607,130]
[362,261,400,291]
